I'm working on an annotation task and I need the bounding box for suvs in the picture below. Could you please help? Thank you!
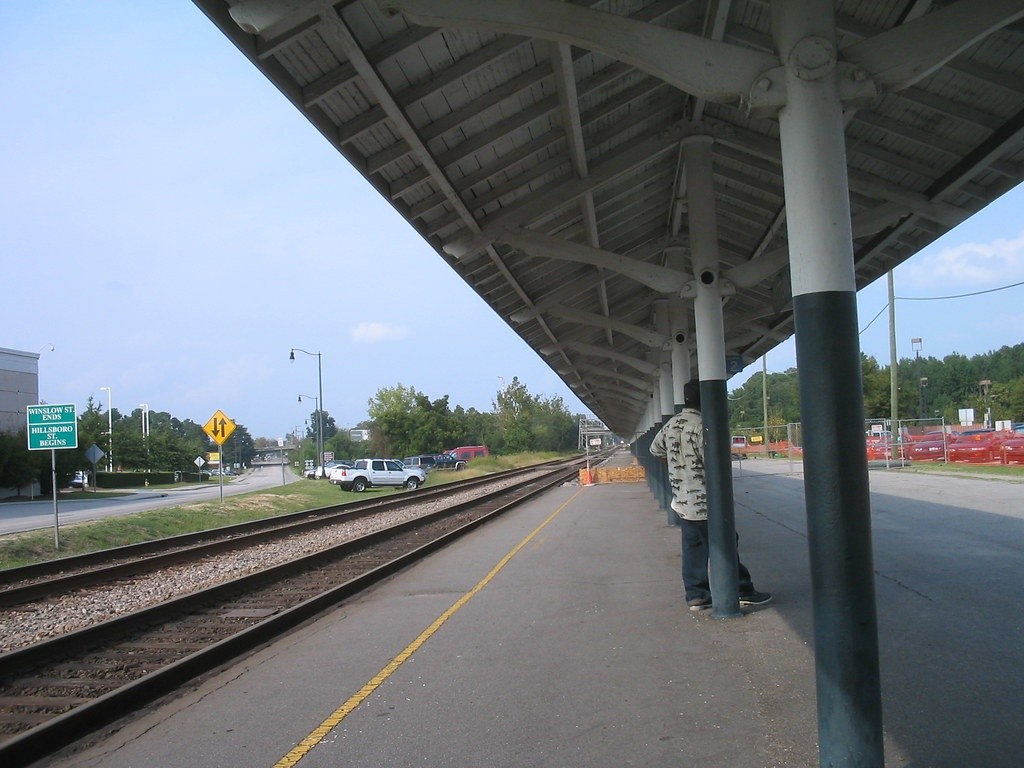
[421,453,467,471]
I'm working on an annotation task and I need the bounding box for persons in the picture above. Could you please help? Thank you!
[647,379,772,612]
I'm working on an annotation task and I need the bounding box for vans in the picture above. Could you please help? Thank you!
[404,456,438,472]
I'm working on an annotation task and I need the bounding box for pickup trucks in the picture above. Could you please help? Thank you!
[330,457,427,493]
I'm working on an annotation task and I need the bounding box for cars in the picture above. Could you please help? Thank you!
[316,460,352,477]
[1000,423,1024,464]
[873,434,912,458]
[946,428,1001,461]
[912,431,958,460]
[866,430,891,457]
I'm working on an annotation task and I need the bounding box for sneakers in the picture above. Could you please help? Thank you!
[739,591,773,605]
[689,597,713,610]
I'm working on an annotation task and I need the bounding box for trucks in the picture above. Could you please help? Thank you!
[449,446,489,469]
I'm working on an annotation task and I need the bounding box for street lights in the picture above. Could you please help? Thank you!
[100,386,113,472]
[296,395,320,471]
[139,402,151,473]
[289,348,326,478]
